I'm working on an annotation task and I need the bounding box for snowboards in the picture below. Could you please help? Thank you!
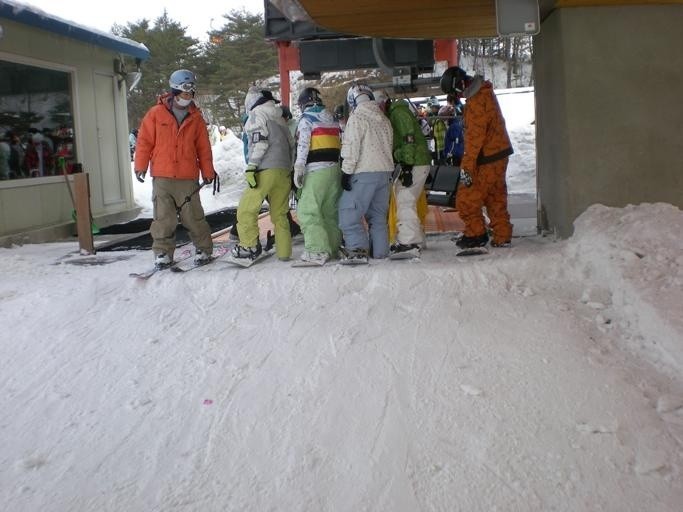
[217,230,491,268]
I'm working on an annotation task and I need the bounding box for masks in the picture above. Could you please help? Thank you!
[174,95,191,107]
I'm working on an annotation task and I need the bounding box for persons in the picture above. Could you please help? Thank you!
[233,66,514,270]
[132,69,217,267]
[0,126,75,179]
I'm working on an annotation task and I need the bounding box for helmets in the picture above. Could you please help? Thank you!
[168,69,197,93]
[409,102,422,117]
[373,89,389,109]
[298,87,323,111]
[439,66,466,95]
[333,104,347,118]
[436,106,456,120]
[347,83,376,109]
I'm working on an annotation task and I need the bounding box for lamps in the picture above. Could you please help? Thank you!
[115,69,143,92]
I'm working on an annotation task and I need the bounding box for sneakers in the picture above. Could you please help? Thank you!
[231,241,263,257]
[455,233,489,248]
[389,243,421,259]
[300,249,329,261]
[229,220,240,240]
[194,248,211,265]
[490,238,512,248]
[154,251,173,267]
[339,248,369,265]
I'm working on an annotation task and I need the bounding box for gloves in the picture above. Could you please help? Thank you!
[444,153,453,167]
[293,164,306,189]
[204,178,212,184]
[341,171,352,191]
[460,168,473,187]
[243,164,259,189]
[136,170,145,183]
[398,164,414,187]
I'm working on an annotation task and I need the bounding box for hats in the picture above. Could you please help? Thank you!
[219,125,226,133]
[428,95,440,108]
[245,87,282,110]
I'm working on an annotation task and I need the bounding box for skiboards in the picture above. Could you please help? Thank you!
[129,239,234,279]
